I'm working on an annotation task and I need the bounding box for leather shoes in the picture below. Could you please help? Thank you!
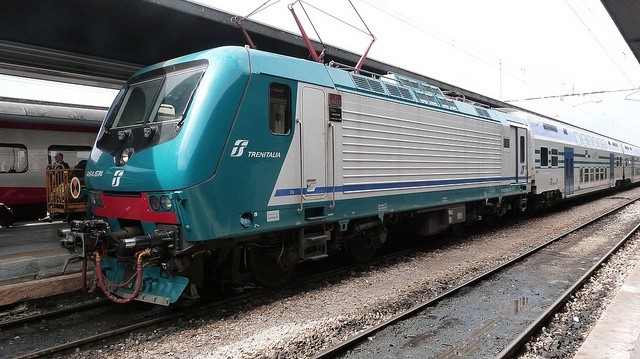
[38,217,52,223]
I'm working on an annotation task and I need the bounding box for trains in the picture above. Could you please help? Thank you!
[0,101,109,226]
[57,45,640,306]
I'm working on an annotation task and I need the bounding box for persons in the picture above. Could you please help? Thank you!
[37,153,71,222]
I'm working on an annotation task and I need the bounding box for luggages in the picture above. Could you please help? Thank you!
[0,203,16,228]
